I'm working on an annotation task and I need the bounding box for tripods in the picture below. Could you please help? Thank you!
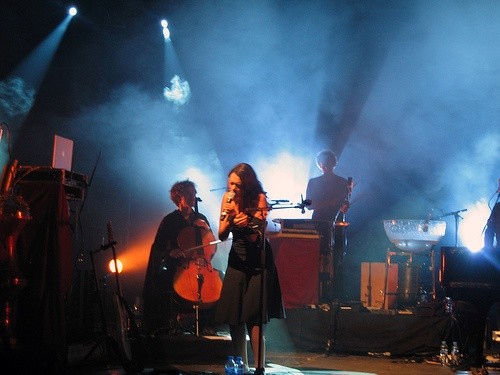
[74,240,118,372]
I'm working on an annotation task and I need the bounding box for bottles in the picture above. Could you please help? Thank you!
[225,355,238,375]
[445,297,452,313]
[440,341,448,354]
[235,357,244,375]
[451,342,459,353]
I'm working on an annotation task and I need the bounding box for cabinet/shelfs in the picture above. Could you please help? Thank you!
[269,233,320,309]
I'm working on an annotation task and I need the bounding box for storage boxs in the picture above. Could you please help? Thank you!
[361,262,398,309]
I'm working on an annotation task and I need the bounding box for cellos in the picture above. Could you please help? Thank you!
[170,197,223,338]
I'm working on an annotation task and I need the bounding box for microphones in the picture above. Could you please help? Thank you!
[220,192,234,221]
[300,200,312,208]
[423,211,432,232]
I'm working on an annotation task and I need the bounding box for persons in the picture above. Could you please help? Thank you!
[213,163,287,375]
[304,150,352,304]
[139,180,225,335]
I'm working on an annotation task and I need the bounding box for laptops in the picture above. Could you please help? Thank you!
[19,135,73,169]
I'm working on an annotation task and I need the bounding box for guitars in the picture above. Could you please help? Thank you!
[107,219,132,363]
